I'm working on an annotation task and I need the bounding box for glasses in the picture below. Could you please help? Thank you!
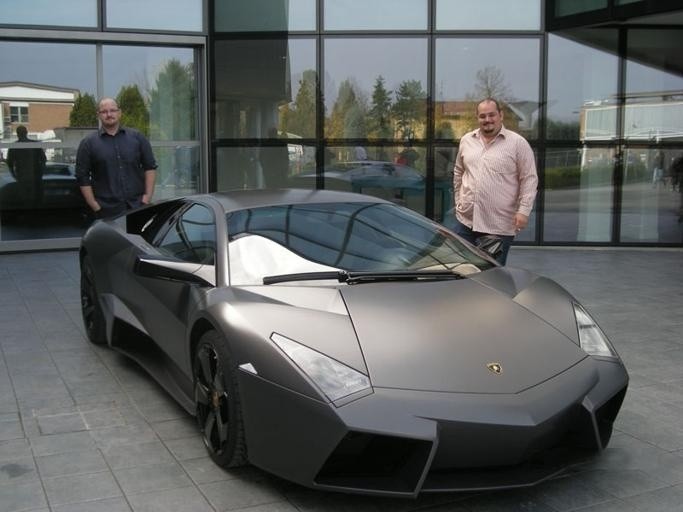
[97,109,120,113]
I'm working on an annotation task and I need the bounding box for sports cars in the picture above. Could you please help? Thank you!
[0,162,81,207]
[298,160,424,189]
[79,189,629,499]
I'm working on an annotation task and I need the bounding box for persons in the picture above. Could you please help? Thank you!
[256,121,448,196]
[449,95,538,269]
[72,97,159,221]
[4,125,49,217]
[650,149,667,190]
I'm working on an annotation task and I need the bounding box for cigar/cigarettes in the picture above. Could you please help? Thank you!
[516,227,520,232]
